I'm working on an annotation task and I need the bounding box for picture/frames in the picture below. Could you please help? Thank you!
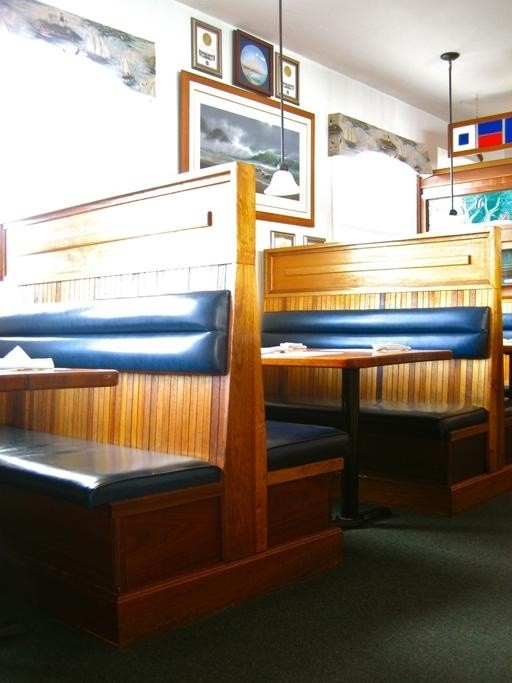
[177,15,317,230]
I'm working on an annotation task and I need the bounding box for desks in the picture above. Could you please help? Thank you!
[261,343,452,532]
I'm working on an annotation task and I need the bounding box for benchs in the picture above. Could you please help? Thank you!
[1,286,231,652]
[267,420,350,591]
[267,305,487,517]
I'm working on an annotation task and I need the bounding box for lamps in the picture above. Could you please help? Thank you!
[260,0,301,198]
